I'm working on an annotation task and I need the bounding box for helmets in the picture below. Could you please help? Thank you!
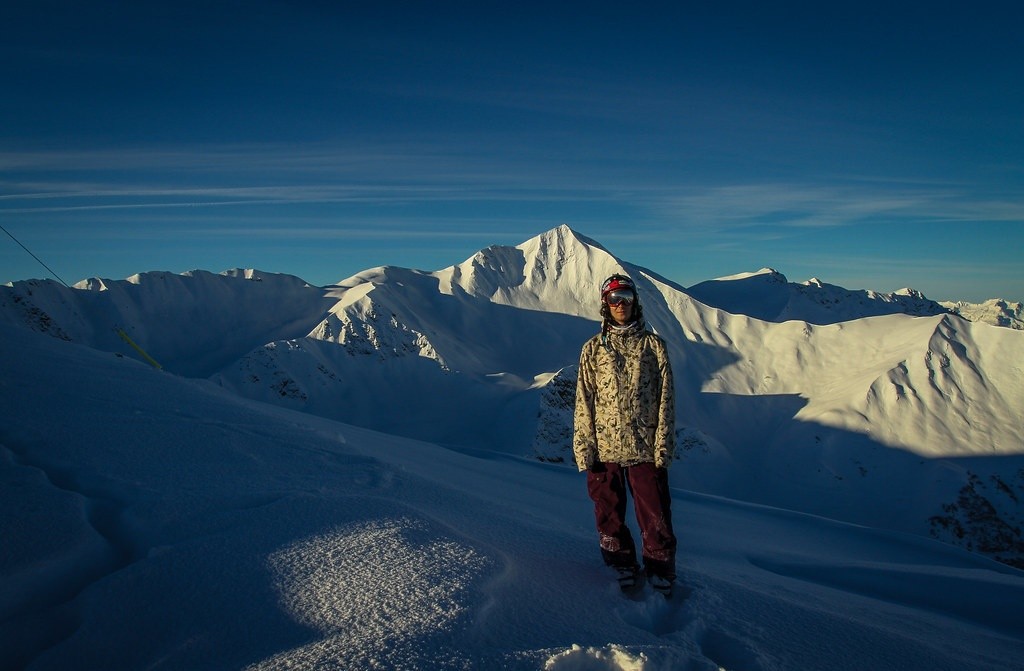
[602,275,638,304]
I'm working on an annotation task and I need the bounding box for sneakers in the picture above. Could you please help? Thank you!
[613,557,641,594]
[643,555,676,599]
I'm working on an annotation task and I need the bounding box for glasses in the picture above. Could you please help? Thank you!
[606,288,634,308]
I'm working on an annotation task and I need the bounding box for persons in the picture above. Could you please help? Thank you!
[571,271,680,600]
